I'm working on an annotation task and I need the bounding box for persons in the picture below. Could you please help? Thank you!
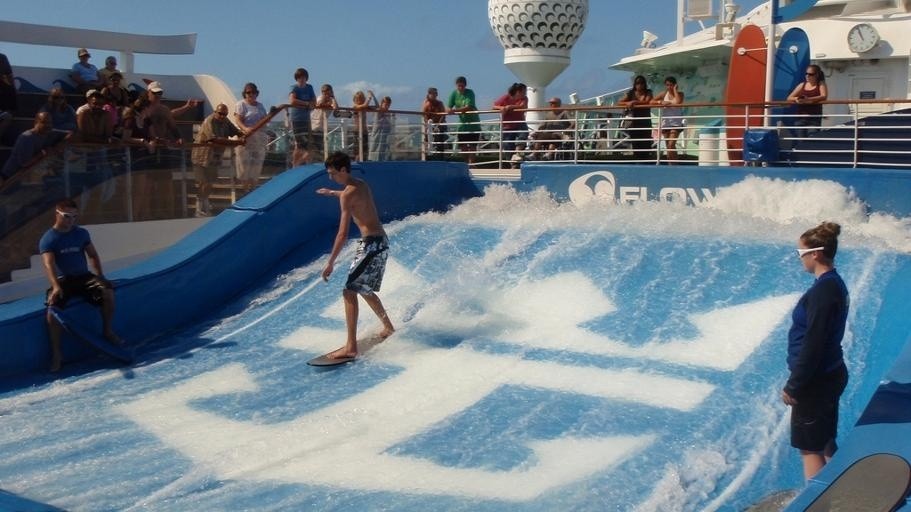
[193,83,276,214]
[787,65,828,142]
[288,68,392,167]
[447,76,482,169]
[781,221,849,479]
[39,200,120,372]
[493,82,529,169]
[618,76,653,164]
[316,152,395,359]
[1,48,198,180]
[524,97,574,161]
[422,87,448,161]
[648,77,684,164]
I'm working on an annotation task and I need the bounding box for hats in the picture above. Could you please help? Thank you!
[547,98,560,103]
[85,89,100,98]
[147,81,164,93]
[77,48,89,58]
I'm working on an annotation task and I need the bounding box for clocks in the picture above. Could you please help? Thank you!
[846,23,880,54]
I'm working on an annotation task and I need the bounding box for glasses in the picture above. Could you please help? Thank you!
[805,73,815,75]
[54,208,80,220]
[218,112,226,116]
[245,91,255,94]
[794,247,824,259]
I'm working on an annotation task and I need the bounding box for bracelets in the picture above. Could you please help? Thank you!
[658,100,661,104]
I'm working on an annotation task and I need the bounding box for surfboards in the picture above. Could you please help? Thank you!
[726,25,767,166]
[804,453,911,511]
[306,337,386,366]
[47,304,134,360]
[771,28,809,167]
